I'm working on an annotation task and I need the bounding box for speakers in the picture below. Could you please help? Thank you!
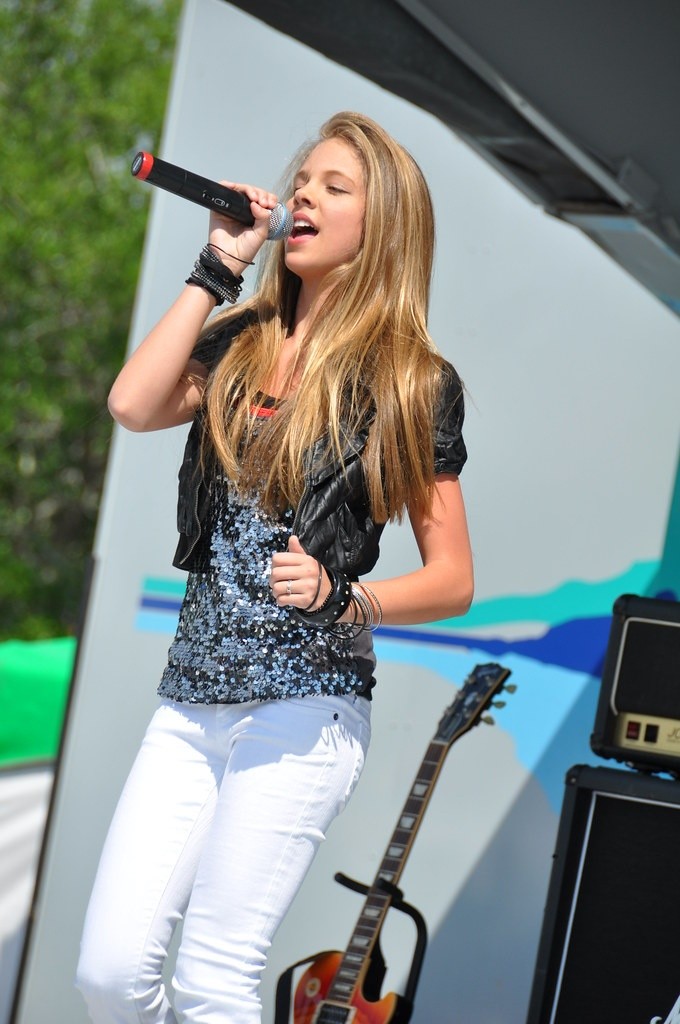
[526,766,680,1024]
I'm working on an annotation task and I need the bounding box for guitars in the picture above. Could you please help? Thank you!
[272,661,517,1024]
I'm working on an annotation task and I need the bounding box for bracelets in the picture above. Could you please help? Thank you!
[294,553,382,640]
[186,243,254,304]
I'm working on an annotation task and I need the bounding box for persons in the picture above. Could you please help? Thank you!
[76,113,475,1024]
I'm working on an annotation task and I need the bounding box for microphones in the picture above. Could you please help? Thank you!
[131,152,294,242]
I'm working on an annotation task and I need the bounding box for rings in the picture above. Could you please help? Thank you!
[286,580,292,594]
